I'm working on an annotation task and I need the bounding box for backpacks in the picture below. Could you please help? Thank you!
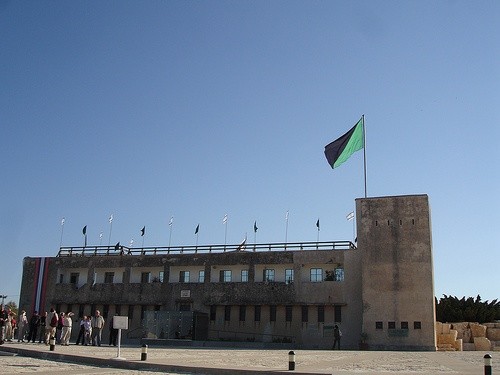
[49,313,58,327]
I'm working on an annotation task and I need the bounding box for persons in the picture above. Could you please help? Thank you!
[28,311,39,343]
[0,305,16,343]
[38,311,47,343]
[109,314,118,346]
[332,324,340,349]
[91,310,105,346]
[61,312,72,346]
[46,307,58,345]
[59,312,65,331]
[76,316,93,345]
[17,310,28,342]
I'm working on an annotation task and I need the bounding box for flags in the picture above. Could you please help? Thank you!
[223,215,227,224]
[324,118,363,169]
[108,216,112,223]
[83,226,86,234]
[141,226,145,236]
[195,224,199,234]
[346,212,354,221]
[254,222,258,232]
[316,220,320,230]
[169,217,173,226]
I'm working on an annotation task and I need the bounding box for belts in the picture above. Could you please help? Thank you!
[62,325,71,327]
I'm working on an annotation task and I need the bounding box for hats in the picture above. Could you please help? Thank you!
[33,311,38,313]
[50,308,55,312]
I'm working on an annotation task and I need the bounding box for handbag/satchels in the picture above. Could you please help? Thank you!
[339,329,343,337]
